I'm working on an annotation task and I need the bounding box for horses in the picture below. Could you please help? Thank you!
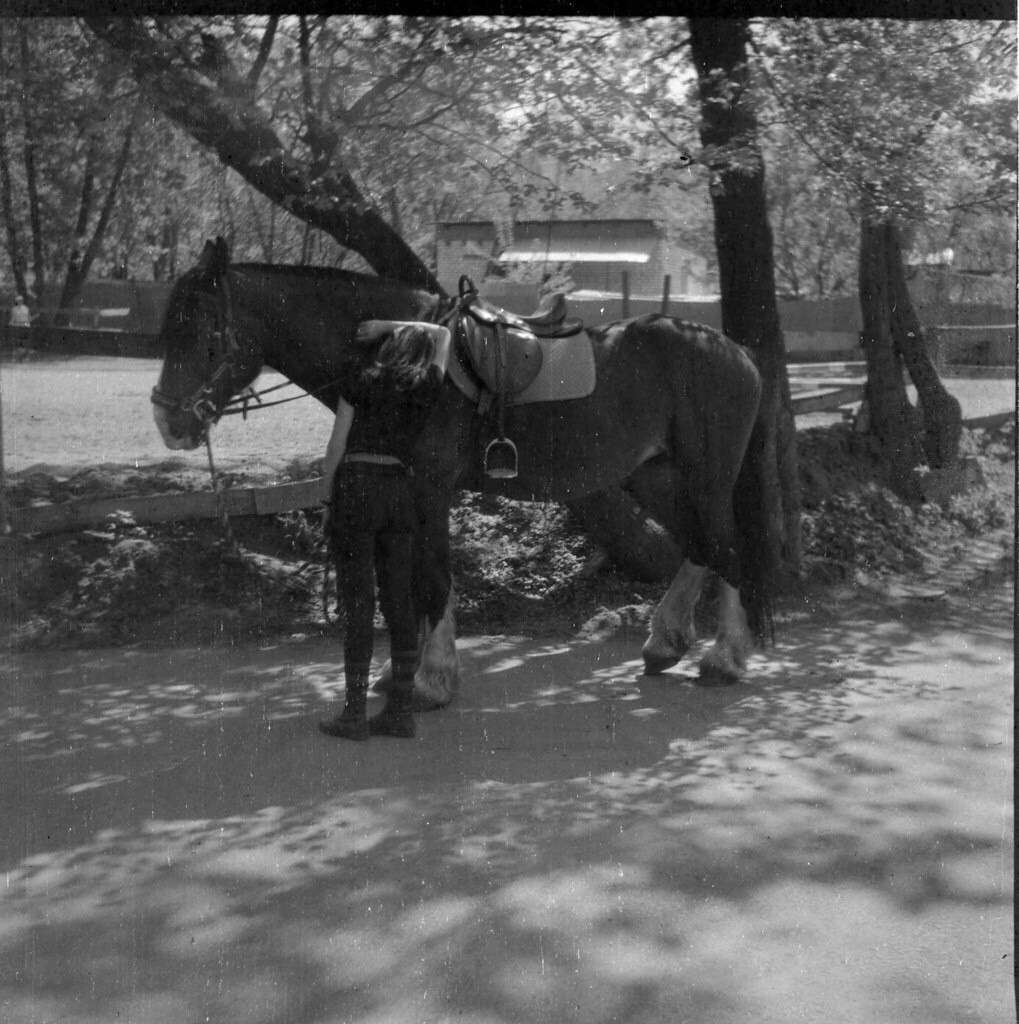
[151,236,778,742]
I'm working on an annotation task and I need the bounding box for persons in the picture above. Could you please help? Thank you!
[319,320,451,742]
[8,295,74,330]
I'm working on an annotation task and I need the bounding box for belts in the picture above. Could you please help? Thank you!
[336,461,406,475]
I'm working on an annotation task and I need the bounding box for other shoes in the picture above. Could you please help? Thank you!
[320,714,368,741]
[368,711,416,737]
